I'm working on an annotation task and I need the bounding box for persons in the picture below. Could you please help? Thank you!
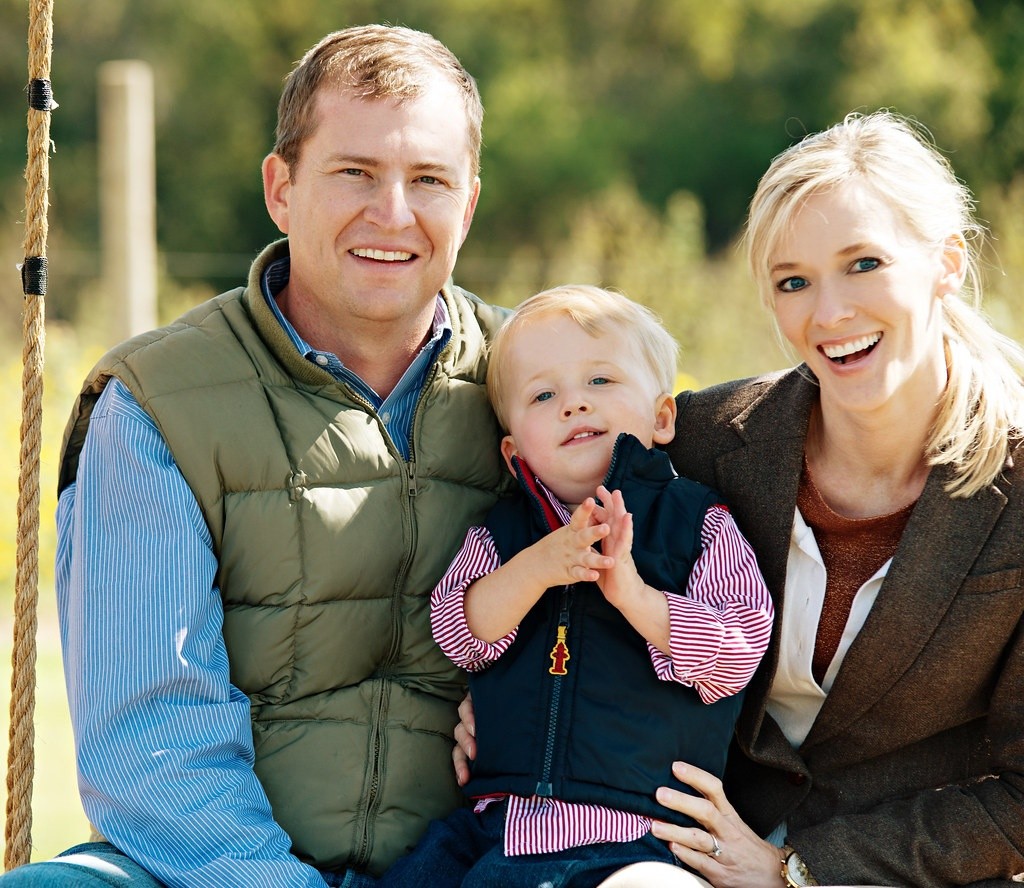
[2,23,519,888]
[452,111,1024,888]
[381,282,775,888]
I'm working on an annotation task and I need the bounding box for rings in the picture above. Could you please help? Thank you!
[706,833,723,858]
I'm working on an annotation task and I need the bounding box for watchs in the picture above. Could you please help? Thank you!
[779,842,817,888]
